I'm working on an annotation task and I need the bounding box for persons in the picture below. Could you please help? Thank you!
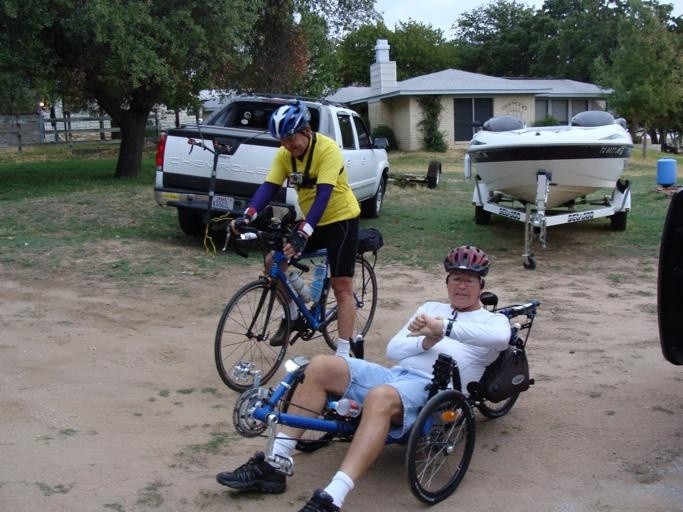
[213,242,513,512]
[226,103,365,358]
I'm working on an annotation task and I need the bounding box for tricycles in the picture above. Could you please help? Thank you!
[222,290,549,512]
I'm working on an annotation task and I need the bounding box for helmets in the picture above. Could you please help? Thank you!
[444,244,491,276]
[268,101,313,140]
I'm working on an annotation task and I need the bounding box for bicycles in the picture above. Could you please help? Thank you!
[207,221,382,394]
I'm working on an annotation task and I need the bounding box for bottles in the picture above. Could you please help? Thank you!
[289,272,311,303]
[311,260,326,304]
[329,398,363,418]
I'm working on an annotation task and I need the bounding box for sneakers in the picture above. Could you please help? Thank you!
[270,311,306,346]
[217,451,290,495]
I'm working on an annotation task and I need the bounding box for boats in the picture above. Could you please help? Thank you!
[452,109,638,272]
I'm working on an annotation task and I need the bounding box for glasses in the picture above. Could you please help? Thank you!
[446,273,482,288]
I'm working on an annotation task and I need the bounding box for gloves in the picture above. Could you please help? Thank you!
[228,205,258,232]
[284,218,315,254]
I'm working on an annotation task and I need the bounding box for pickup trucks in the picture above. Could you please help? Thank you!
[148,93,394,244]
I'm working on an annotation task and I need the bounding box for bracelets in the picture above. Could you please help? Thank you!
[442,316,455,337]
